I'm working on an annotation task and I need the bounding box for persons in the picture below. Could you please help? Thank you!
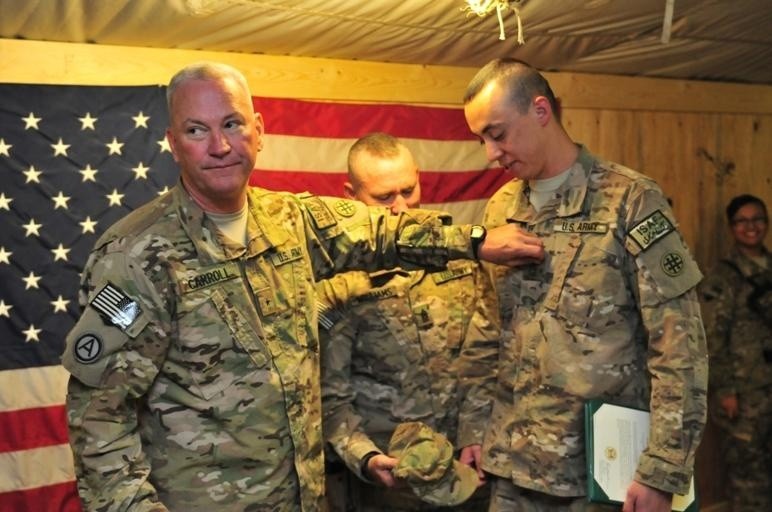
[701,194,772,511]
[322,130,476,512]
[60,62,544,511]
[452,57,708,512]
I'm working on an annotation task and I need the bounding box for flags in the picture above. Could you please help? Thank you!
[0,81,514,511]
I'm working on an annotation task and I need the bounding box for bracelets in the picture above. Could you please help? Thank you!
[361,452,379,481]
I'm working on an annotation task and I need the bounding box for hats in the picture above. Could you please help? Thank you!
[386,420,480,509]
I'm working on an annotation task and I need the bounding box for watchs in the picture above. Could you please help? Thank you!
[471,222,488,261]
